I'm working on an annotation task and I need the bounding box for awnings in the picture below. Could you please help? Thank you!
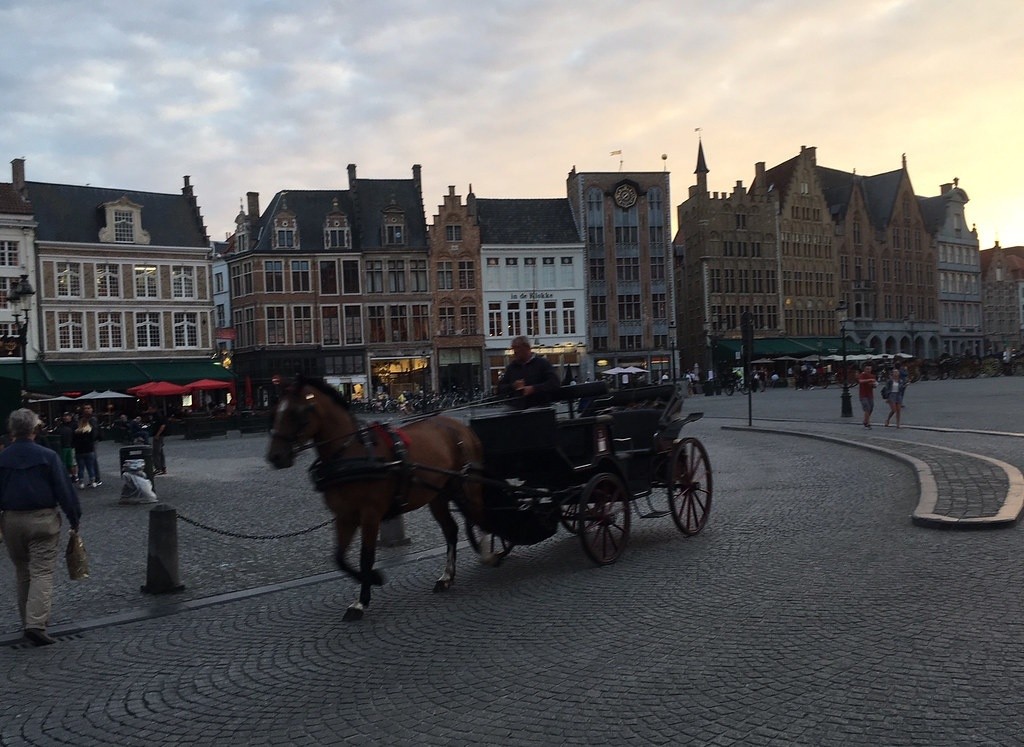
[0,360,238,392]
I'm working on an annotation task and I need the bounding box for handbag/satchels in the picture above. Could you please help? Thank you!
[66,531,88,580]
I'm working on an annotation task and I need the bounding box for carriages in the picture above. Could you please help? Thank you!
[265,369,714,621]
[799,350,1024,392]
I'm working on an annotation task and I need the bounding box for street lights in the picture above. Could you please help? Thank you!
[702,311,728,378]
[668,320,679,383]
[836,300,855,418]
[212,362,241,413]
[4,272,34,407]
[904,308,914,357]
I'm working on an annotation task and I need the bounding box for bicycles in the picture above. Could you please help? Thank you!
[348,384,499,413]
[30,403,227,440]
[726,370,752,395]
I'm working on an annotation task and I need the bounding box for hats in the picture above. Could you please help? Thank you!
[63,411,71,417]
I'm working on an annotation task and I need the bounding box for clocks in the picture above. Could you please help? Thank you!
[615,183,637,208]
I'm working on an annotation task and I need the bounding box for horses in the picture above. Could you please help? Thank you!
[264,369,493,623]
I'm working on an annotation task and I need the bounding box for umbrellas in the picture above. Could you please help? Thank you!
[28,379,233,405]
[749,352,916,364]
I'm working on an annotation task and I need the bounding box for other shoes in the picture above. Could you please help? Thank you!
[154,468,167,475]
[24,628,55,645]
[87,480,102,487]
[79,484,85,491]
[91,482,97,487]
[70,474,79,482]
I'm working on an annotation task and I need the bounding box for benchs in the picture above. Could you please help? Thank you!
[551,380,614,483]
[577,409,683,482]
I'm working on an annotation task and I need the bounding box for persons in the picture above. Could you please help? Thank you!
[884,369,903,429]
[496,335,562,410]
[0,407,83,646]
[569,364,825,394]
[61,404,103,491]
[147,400,167,476]
[856,365,878,430]
